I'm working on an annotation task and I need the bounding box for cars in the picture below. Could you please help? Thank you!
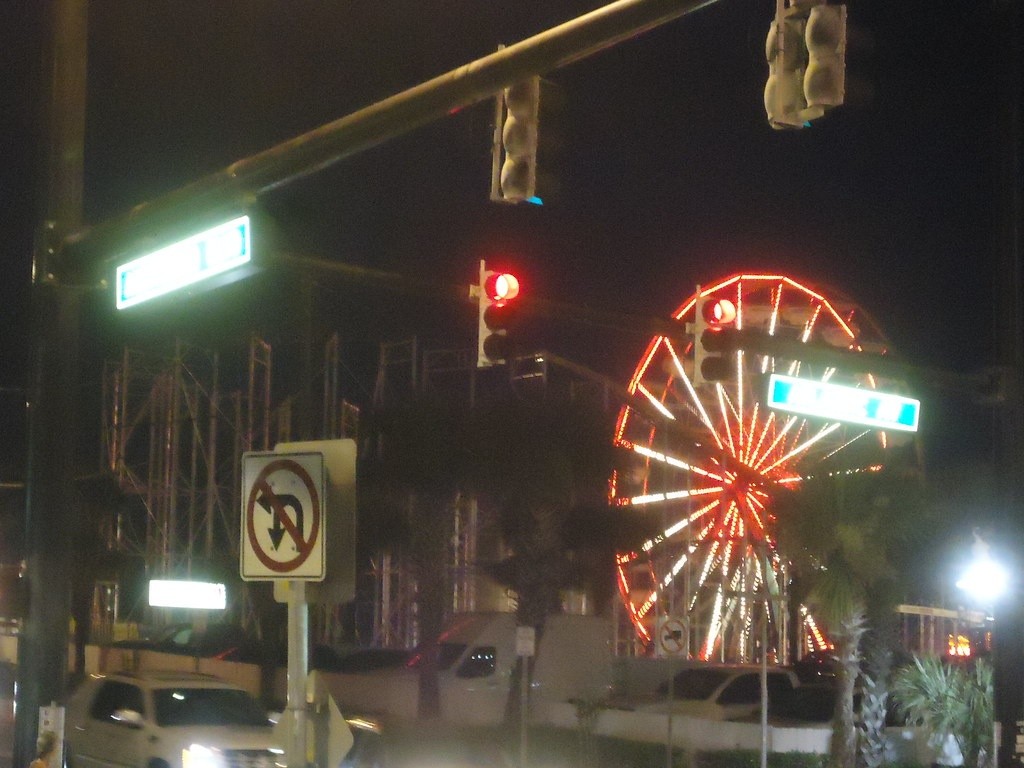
[52,667,284,768]
[111,619,246,661]
[644,646,935,728]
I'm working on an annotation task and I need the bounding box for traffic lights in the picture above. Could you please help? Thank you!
[946,632,976,665]
[476,271,521,367]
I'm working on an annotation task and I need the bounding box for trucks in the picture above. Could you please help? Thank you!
[382,609,617,738]
